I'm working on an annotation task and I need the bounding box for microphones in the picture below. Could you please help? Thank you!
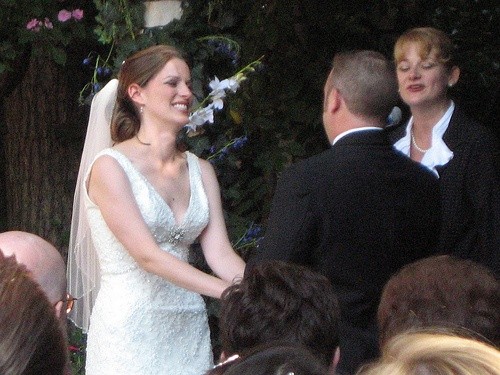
[385,106,402,127]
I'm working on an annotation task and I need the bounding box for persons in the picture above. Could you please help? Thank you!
[375,26,500,271]
[0,230,500,375]
[82,43,246,375]
[243,50,443,375]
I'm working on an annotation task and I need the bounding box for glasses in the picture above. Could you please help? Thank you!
[64,294,75,313]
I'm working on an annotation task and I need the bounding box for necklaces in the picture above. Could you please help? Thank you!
[130,143,187,201]
[410,125,433,153]
